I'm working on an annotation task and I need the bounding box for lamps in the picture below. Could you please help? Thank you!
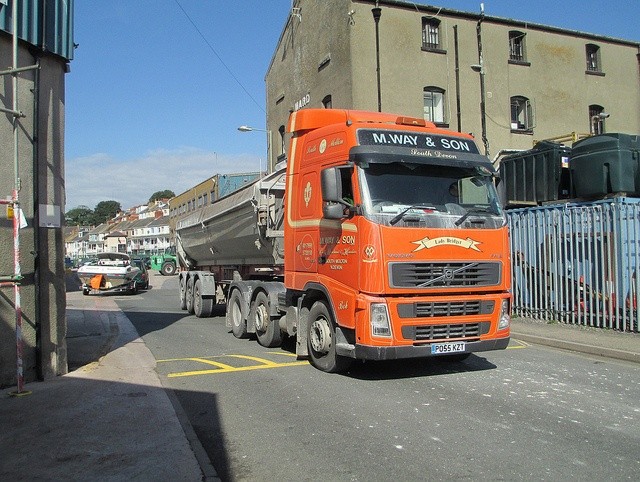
[238,126,269,132]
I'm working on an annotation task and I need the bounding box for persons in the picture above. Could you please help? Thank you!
[342,180,378,214]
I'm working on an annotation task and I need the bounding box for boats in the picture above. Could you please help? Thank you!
[75,251,141,295]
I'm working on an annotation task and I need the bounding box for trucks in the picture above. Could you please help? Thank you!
[168,105,513,375]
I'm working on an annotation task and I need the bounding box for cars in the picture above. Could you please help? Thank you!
[132,257,150,290]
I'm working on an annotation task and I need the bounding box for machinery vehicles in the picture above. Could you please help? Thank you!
[150,245,177,277]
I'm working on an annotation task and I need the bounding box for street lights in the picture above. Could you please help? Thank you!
[238,125,273,175]
